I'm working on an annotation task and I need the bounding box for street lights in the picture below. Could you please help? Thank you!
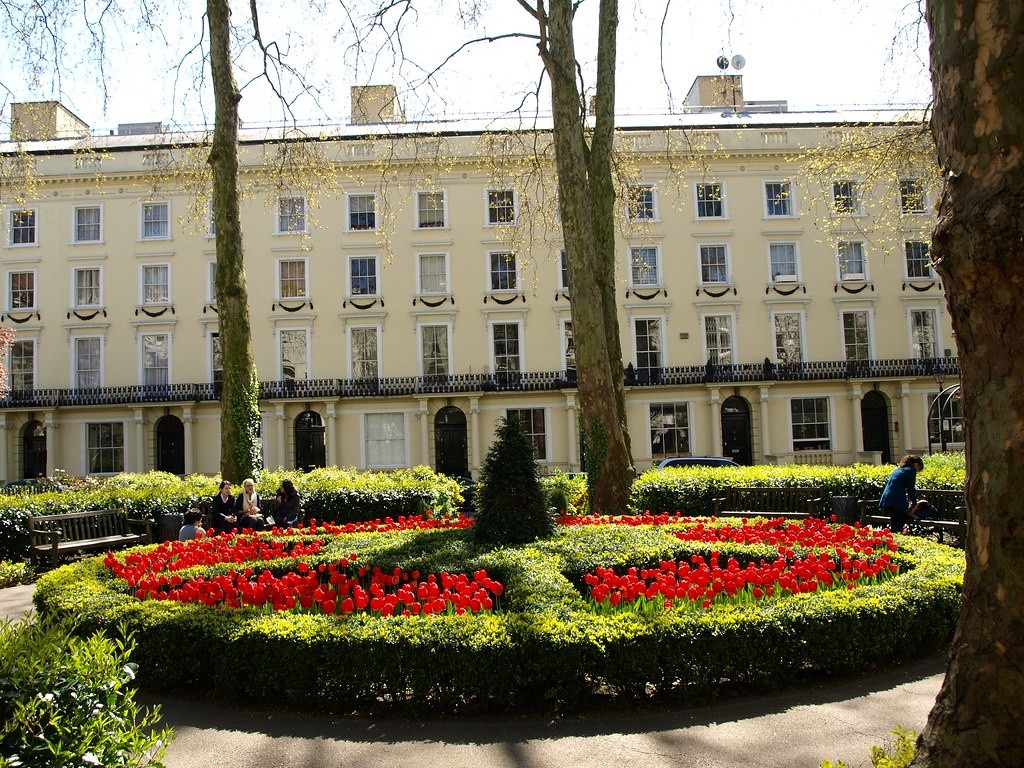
[933,363,947,455]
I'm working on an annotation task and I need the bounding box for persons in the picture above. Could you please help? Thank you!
[212,481,239,533]
[877,455,924,532]
[179,508,206,541]
[236,478,264,532]
[275,479,300,528]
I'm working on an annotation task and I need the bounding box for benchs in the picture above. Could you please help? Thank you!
[205,500,306,535]
[857,482,968,550]
[711,485,824,520]
[28,503,152,569]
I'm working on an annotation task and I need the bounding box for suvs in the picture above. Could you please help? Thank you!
[657,457,740,468]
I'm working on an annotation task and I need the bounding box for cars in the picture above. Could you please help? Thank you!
[0,478,66,494]
[449,478,479,512]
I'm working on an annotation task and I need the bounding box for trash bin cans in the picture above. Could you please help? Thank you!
[157,513,184,542]
[830,496,858,524]
